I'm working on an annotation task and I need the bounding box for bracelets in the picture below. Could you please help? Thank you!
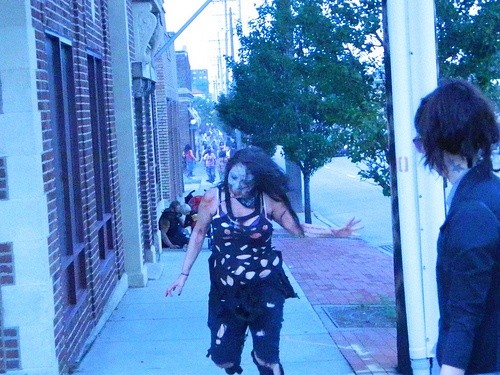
[180,272,188,277]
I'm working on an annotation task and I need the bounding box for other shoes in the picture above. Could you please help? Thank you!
[225,344,244,375]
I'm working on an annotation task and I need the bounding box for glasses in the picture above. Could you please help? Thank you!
[413,136,425,154]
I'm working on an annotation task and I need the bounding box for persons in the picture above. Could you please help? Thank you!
[178,203,198,229]
[202,122,238,179]
[411,81,500,375]
[164,146,366,375]
[182,144,199,179]
[203,147,217,183]
[158,200,190,250]
